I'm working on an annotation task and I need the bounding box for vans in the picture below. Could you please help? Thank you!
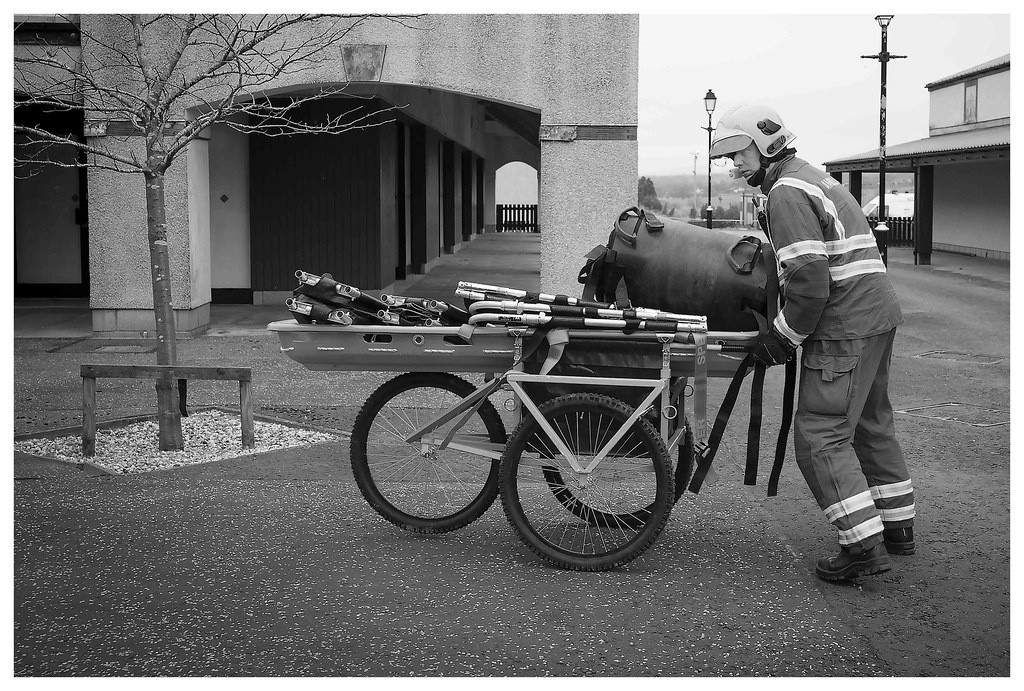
[862,193,914,220]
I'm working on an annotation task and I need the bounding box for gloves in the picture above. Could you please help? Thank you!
[745,330,795,379]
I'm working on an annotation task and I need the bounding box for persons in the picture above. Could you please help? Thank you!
[710,103,917,580]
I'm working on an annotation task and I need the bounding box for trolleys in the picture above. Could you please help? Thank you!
[265,319,793,571]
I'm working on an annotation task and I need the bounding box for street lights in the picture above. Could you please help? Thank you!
[701,89,717,229]
[861,15,907,268]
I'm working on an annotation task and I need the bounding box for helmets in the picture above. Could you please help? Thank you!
[710,103,797,157]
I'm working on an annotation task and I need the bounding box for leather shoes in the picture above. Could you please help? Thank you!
[882,526,916,556]
[815,544,892,580]
